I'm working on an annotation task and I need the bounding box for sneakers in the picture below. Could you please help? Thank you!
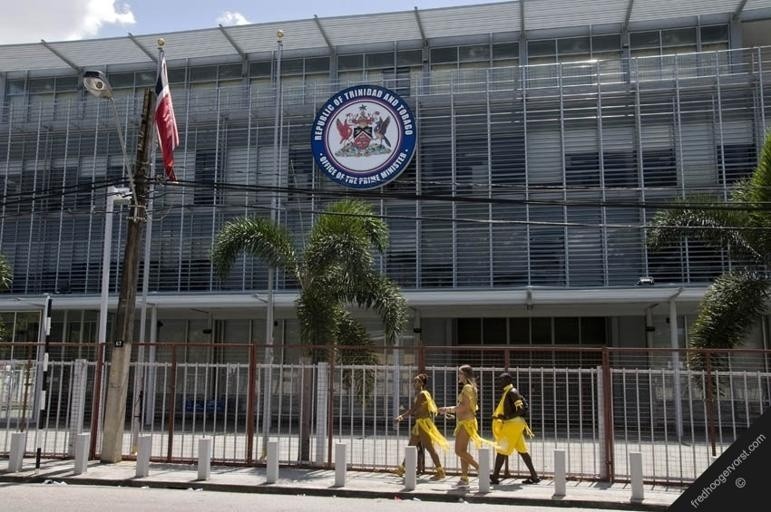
[490,474,540,484]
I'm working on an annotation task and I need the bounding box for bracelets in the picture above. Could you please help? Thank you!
[446,406,455,414]
[397,414,403,422]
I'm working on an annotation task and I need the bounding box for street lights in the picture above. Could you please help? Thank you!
[82,69,138,206]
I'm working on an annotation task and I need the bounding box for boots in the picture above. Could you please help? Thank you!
[429,467,470,489]
[391,466,405,476]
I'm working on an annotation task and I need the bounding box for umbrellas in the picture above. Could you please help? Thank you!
[151,51,179,182]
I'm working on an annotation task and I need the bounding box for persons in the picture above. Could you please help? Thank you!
[438,364,494,488]
[489,373,541,485]
[389,374,445,480]
